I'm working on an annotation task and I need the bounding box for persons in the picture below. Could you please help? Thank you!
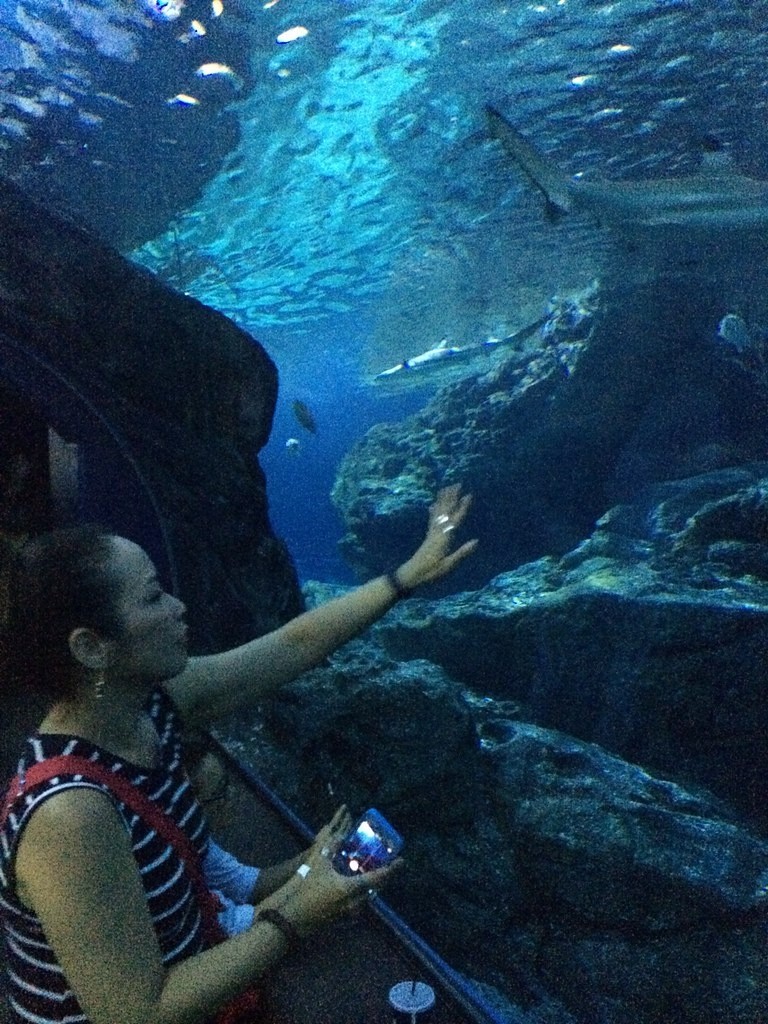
[175,733,355,943]
[1,484,483,1024]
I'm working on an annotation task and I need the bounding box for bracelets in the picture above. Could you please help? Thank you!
[257,906,306,965]
[294,864,311,881]
[296,849,310,866]
[380,561,411,599]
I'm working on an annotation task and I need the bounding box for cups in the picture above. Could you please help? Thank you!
[388,981,435,1024]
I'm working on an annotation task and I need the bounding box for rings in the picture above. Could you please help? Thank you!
[365,885,377,901]
[434,512,456,538]
[318,847,335,860]
[354,875,371,888]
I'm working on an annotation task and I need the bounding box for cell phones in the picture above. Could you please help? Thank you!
[333,809,403,901]
